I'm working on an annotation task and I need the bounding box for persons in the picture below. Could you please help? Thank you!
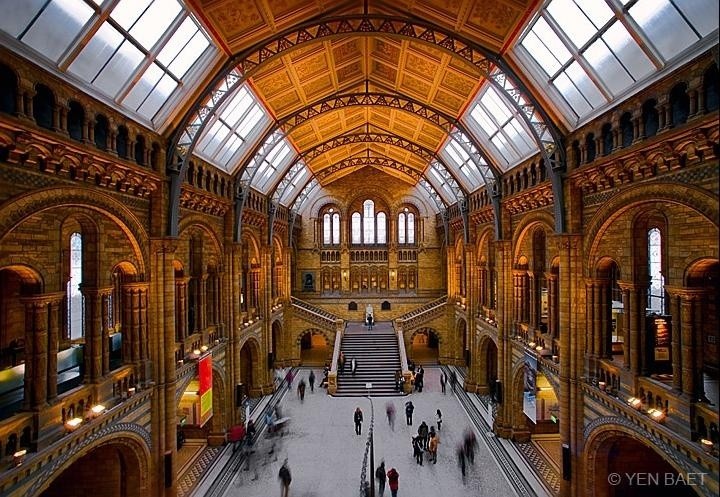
[231,311,480,497]
[365,303,375,322]
